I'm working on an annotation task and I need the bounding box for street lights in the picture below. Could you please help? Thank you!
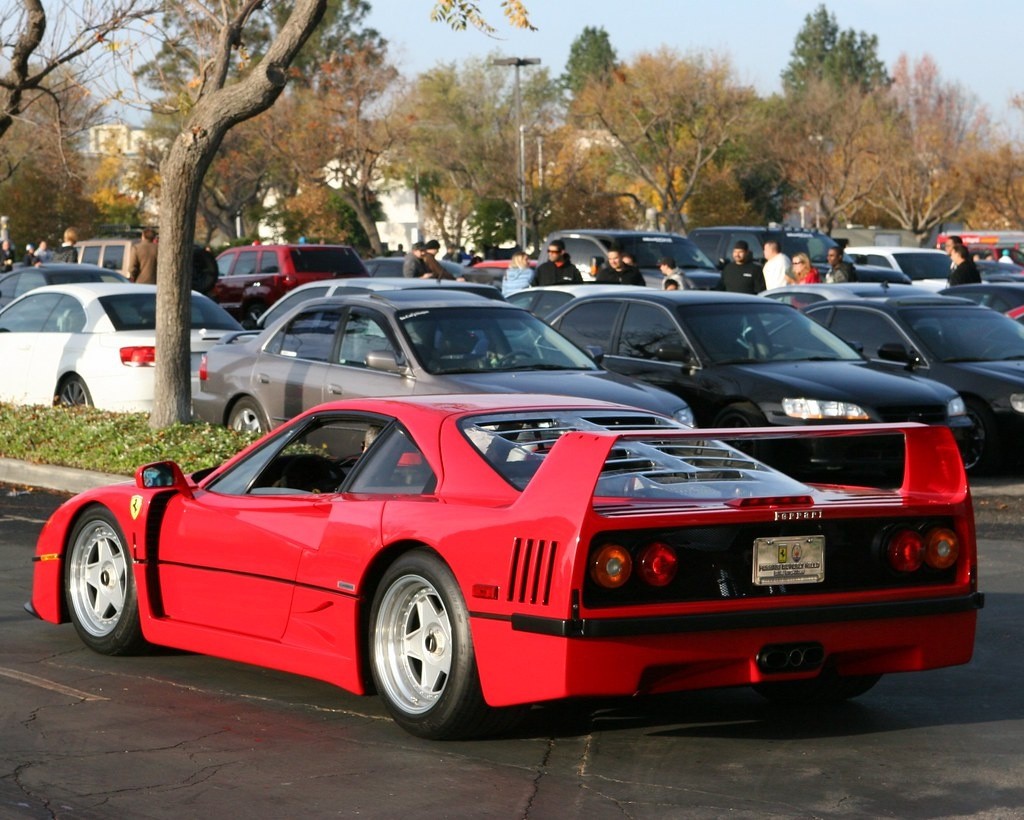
[492,58,542,247]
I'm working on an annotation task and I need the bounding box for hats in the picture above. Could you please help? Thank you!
[733,240,749,249]
[1002,249,1010,256]
[412,241,426,250]
[425,239,440,249]
[25,243,35,251]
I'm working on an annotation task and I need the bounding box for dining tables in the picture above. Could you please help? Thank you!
[352,334,392,362]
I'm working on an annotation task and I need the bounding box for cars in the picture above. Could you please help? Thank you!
[0,263,250,419]
[507,286,985,488]
[359,258,537,290]
[843,247,1024,326]
[251,276,663,337]
[190,287,695,445]
[733,294,1024,473]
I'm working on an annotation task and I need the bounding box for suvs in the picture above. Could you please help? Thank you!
[209,244,369,324]
[688,226,845,284]
[821,224,918,247]
[70,225,147,281]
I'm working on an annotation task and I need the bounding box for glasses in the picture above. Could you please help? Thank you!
[547,250,559,254]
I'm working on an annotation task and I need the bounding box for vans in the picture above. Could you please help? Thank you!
[936,230,1024,254]
[536,230,722,292]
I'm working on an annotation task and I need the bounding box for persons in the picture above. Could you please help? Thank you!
[252,239,261,247]
[192,242,219,296]
[825,246,856,283]
[296,234,327,246]
[532,240,585,286]
[787,252,823,308]
[364,238,483,282]
[50,227,78,264]
[502,253,534,300]
[718,241,767,295]
[946,235,1016,287]
[129,228,159,285]
[359,425,383,459]
[0,240,54,273]
[761,242,791,293]
[656,257,690,292]
[596,250,647,287]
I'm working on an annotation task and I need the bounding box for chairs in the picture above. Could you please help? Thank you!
[440,327,479,355]
[918,327,943,352]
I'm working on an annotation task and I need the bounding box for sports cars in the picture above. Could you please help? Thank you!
[25,393,987,740]
